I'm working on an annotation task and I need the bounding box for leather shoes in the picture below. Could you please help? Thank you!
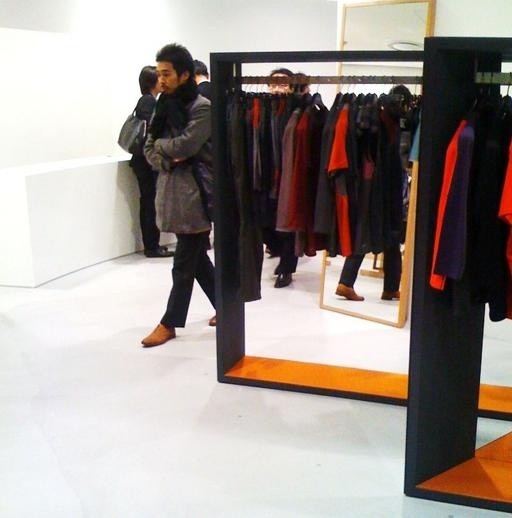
[209,316,217,326]
[334,284,364,300]
[141,324,175,347]
[145,246,174,257]
[382,290,400,300]
[274,264,291,288]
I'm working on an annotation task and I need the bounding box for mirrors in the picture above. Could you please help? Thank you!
[319,0,436,329]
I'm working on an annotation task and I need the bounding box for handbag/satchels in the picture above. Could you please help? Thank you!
[118,115,147,155]
[193,162,215,221]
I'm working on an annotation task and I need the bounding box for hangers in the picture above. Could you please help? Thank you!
[468,69,512,120]
[228,74,423,114]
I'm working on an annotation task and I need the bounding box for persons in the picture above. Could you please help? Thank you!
[333,83,413,303]
[291,73,311,97]
[190,59,211,100]
[129,64,176,258]
[265,67,299,289]
[139,40,219,347]
